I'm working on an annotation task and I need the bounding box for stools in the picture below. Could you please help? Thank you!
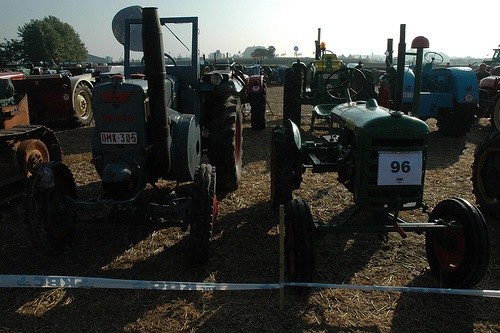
[425,68,451,93]
[309,104,338,141]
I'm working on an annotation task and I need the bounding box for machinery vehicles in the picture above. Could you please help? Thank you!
[8,27,500,220]
[1,72,63,209]
[271,23,492,295]
[21,5,244,261]
